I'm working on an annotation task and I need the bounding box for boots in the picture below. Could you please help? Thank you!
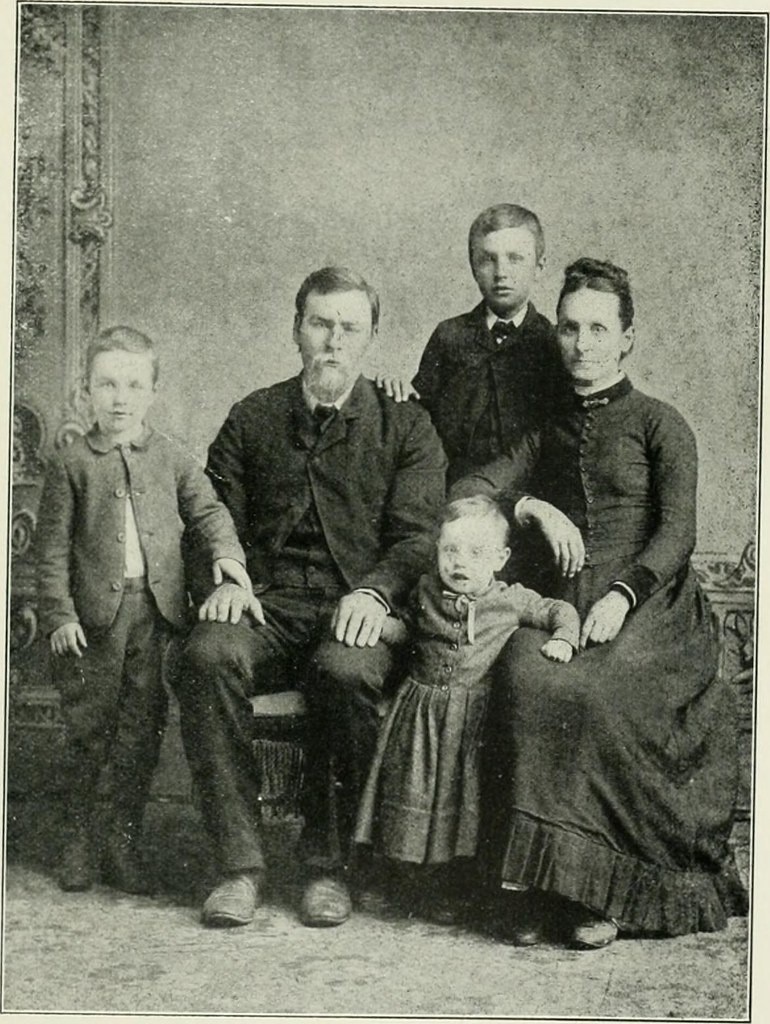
[102,804,155,894]
[56,794,95,892]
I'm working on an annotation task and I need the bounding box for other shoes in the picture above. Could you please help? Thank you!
[424,890,469,925]
[357,884,401,913]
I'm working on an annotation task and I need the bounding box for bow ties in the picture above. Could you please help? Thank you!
[492,321,515,336]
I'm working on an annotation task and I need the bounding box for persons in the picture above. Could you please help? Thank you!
[35,325,250,894]
[353,495,579,923]
[450,256,746,950]
[376,204,568,488]
[165,265,447,926]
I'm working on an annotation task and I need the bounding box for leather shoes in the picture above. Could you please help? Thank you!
[300,876,351,926]
[512,913,552,945]
[202,871,265,926]
[567,920,619,947]
